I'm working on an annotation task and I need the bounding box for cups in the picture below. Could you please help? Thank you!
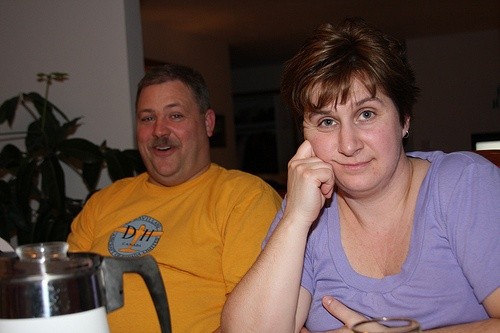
[351,317,422,333]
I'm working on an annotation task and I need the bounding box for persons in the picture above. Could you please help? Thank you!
[220,18,500,333]
[67,64,284,333]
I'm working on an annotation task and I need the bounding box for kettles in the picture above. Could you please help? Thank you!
[0,242,172,333]
